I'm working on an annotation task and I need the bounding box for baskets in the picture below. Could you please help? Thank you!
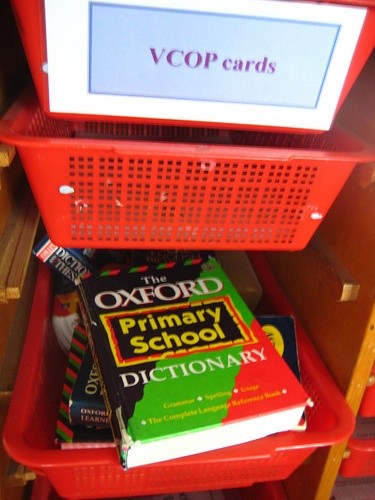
[2,250,352,500]
[1,95,375,251]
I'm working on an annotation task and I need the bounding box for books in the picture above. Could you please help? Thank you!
[33,236,308,470]
